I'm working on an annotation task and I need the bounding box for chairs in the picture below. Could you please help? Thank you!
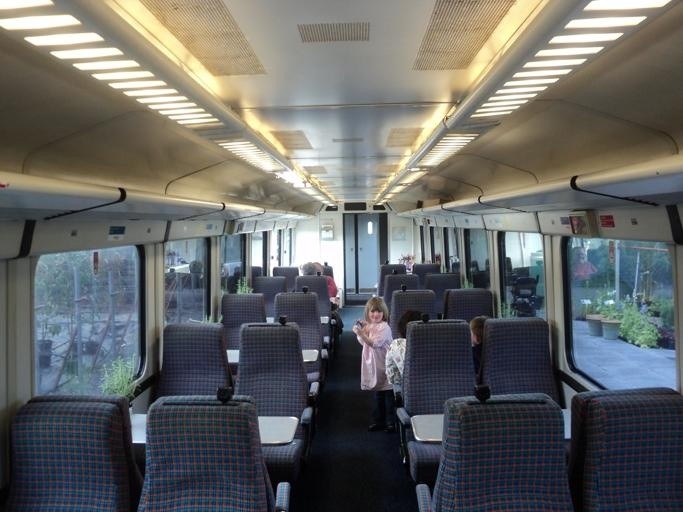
[196,260,339,396]
[136,395,293,509]
[481,319,564,406]
[450,253,540,319]
[416,392,566,511]
[154,323,232,395]
[3,394,131,510]
[570,386,682,512]
[399,322,478,467]
[378,263,543,417]
[232,326,313,482]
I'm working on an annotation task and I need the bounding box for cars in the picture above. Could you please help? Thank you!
[528,248,544,265]
[165,256,190,273]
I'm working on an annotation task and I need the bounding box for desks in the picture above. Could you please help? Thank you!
[126,411,297,446]
[410,408,571,442]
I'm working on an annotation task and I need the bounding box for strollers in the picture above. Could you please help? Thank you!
[510,272,542,317]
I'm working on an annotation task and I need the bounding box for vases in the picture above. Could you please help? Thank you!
[600,319,622,341]
[586,315,602,337]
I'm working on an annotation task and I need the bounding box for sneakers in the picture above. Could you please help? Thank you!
[368,423,393,432]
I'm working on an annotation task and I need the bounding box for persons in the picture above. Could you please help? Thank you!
[352,296,394,430]
[300,262,339,310]
[470,315,488,374]
[385,311,422,407]
